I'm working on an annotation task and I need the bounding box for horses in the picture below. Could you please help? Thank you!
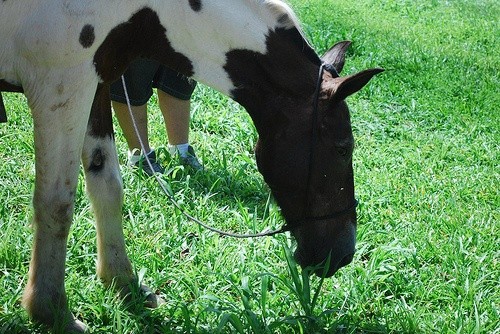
[0,1,385,334]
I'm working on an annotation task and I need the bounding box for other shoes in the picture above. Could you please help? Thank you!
[181,145,203,172]
[126,151,169,181]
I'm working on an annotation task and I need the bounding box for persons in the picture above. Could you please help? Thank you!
[107,56,210,185]
